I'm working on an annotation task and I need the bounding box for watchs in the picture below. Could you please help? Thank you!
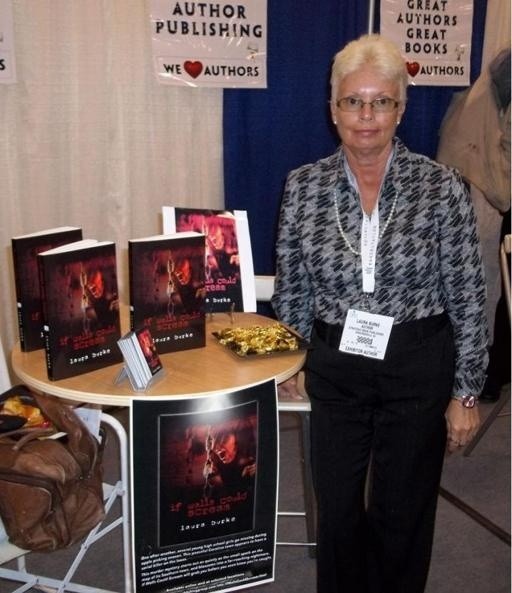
[451,392,482,408]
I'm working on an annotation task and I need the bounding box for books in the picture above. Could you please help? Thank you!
[10,227,210,383]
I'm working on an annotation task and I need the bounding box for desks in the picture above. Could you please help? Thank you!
[12,311,307,593]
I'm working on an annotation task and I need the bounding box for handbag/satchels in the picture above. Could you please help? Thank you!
[1,384,108,553]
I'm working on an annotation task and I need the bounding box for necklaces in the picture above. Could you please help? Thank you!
[334,143,403,258]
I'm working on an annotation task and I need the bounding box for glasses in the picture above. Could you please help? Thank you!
[336,95,401,115]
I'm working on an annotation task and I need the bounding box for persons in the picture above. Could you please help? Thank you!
[437,43,511,404]
[271,33,493,590]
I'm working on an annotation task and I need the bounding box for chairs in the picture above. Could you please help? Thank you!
[1,337,134,593]
[254,275,318,559]
